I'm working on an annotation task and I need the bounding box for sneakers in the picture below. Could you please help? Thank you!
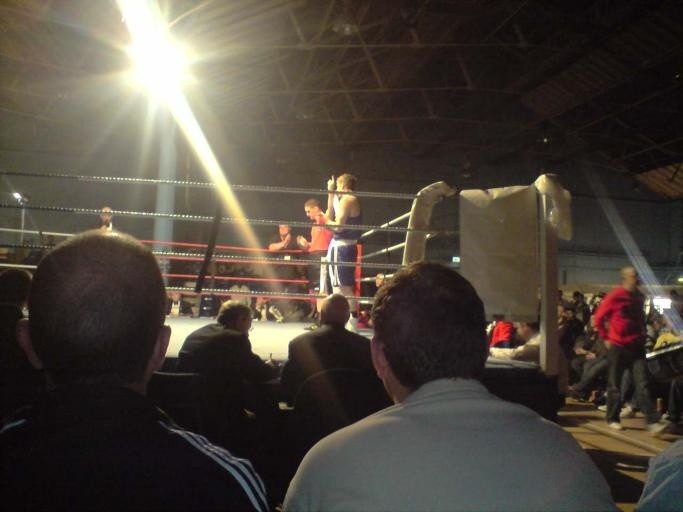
[303,311,321,331]
[344,315,359,334]
[570,383,683,438]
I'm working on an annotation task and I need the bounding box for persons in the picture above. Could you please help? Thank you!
[268,220,308,319]
[296,175,334,321]
[280,261,622,512]
[478,263,683,438]
[314,172,363,336]
[632,439,683,511]
[88,206,120,233]
